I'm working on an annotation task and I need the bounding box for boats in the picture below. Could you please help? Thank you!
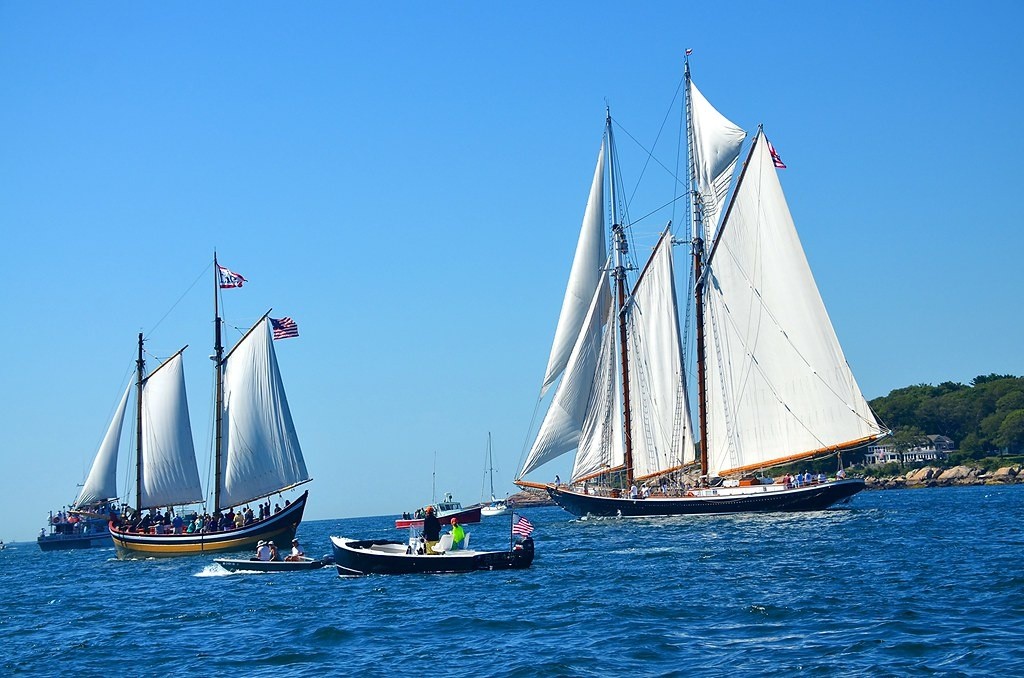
[329,512,535,580]
[34,461,119,552]
[211,554,336,573]
[395,450,482,529]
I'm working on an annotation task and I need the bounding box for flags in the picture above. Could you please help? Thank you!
[767,140,786,168]
[271,316,299,340]
[512,514,534,537]
[686,48,693,56]
[218,265,247,288]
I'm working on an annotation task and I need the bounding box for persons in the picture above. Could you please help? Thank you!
[805,469,812,485]
[674,480,677,487]
[250,540,282,561]
[630,483,651,499]
[654,484,666,493]
[828,470,845,482]
[688,484,692,489]
[284,539,304,561]
[694,480,699,488]
[584,480,588,494]
[555,475,560,486]
[797,471,803,486]
[403,506,465,555]
[818,470,827,483]
[785,473,798,488]
[38,498,292,537]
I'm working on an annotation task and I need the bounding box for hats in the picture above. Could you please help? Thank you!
[268,540,274,544]
[427,507,433,513]
[256,540,267,548]
[451,518,458,523]
[236,510,240,514]
[291,539,297,543]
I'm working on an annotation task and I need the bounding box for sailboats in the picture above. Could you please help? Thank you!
[511,45,895,524]
[479,431,512,517]
[64,249,314,564]
